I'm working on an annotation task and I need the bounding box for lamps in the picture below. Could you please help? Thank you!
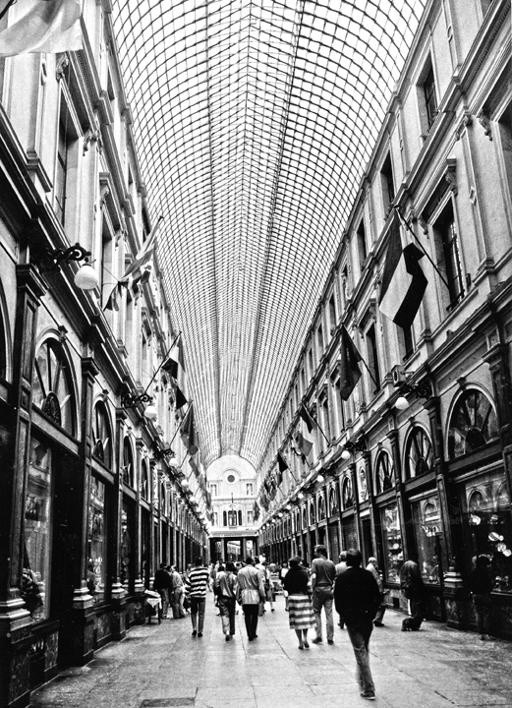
[286,497,301,509]
[117,391,157,419]
[171,472,187,486]
[156,449,178,468]
[396,382,431,411]
[341,441,363,459]
[298,487,313,499]
[43,240,99,289]
[186,489,209,530]
[317,466,339,483]
[254,508,290,536]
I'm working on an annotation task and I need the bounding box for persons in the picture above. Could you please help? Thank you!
[333,547,382,701]
[367,556,389,627]
[151,544,350,651]
[400,555,426,631]
[470,556,496,641]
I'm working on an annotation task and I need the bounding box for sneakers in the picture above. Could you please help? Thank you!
[360,689,376,700]
